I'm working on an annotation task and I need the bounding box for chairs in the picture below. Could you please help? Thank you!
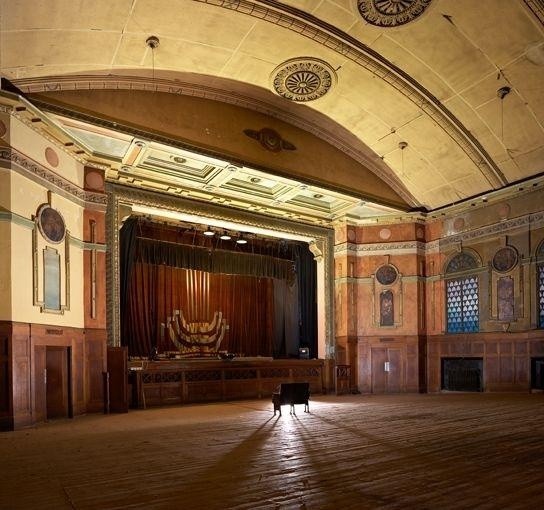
[273,382,311,416]
